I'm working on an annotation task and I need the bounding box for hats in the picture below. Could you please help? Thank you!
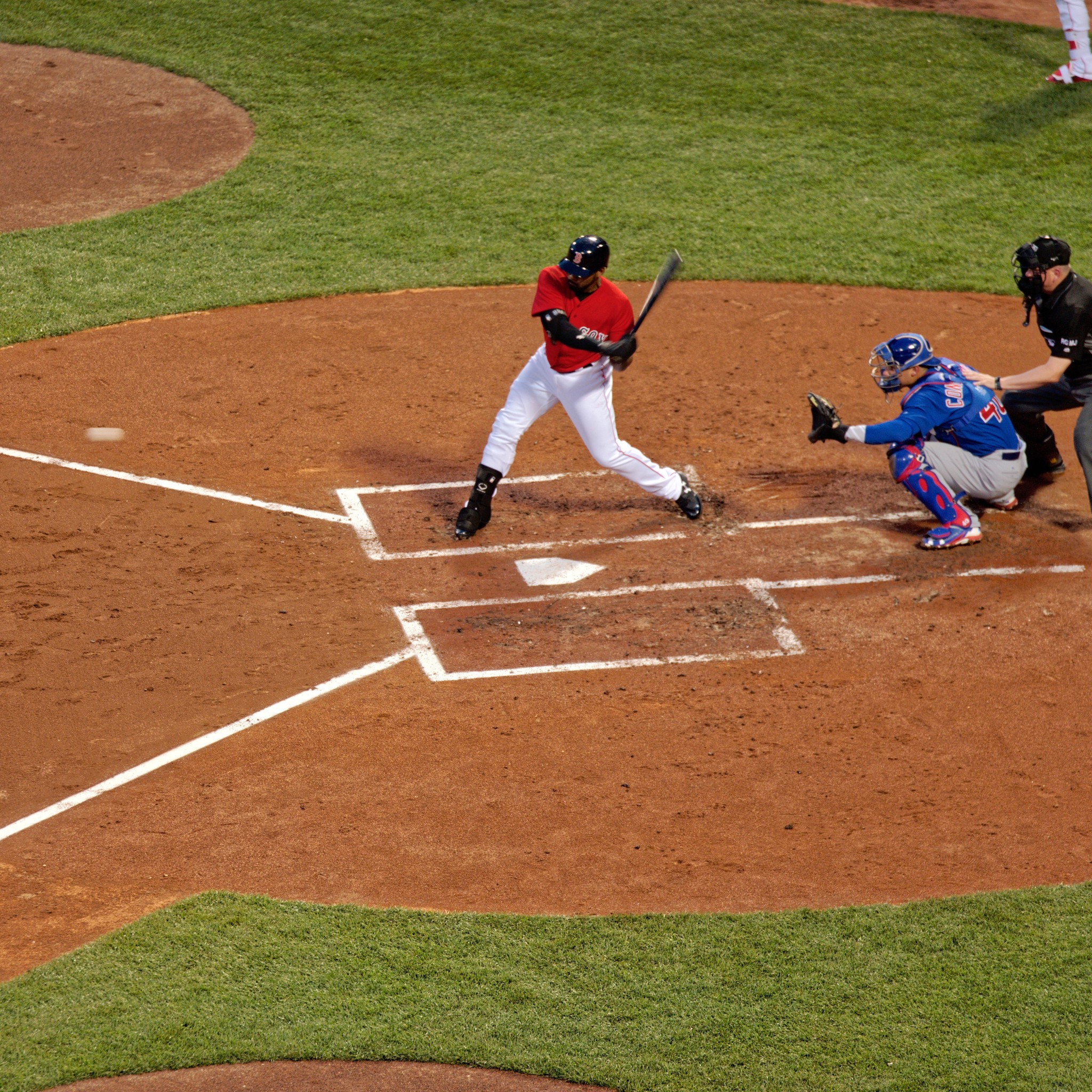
[1031,237,1071,269]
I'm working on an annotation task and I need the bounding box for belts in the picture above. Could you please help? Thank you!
[1001,452,1019,461]
[567,364,592,374]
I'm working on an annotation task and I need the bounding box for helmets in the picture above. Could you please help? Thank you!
[887,333,942,367]
[559,235,609,278]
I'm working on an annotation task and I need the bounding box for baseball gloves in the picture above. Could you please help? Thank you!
[806,391,842,444]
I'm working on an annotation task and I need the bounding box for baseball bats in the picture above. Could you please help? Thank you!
[616,249,683,358]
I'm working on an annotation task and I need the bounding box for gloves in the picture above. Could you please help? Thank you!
[602,333,637,364]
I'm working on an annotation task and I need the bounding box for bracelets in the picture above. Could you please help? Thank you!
[995,377,1002,390]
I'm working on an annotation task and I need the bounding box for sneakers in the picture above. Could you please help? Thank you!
[676,473,702,521]
[921,525,983,548]
[453,505,491,537]
[968,488,1018,510]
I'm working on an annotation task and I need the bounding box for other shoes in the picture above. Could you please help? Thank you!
[1021,461,1066,480]
[1056,76,1092,84]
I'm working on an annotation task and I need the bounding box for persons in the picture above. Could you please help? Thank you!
[805,329,1029,551]
[957,236,1092,505]
[1044,0,1092,84]
[454,234,704,542]
